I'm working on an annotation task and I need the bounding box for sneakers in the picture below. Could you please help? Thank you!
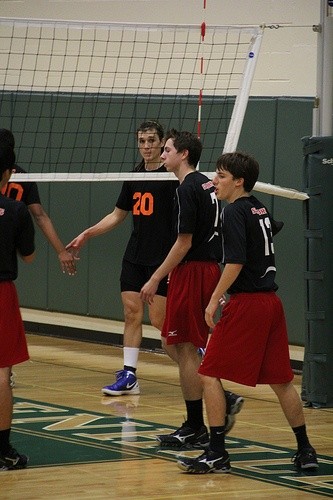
[224,390,244,435]
[0,445,29,471]
[292,446,319,469]
[176,448,233,475]
[101,369,141,395]
[157,420,210,450]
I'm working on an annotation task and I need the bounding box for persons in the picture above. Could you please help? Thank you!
[0,128,80,388]
[176,153,319,473]
[140,127,245,448]
[0,143,37,469]
[66,121,206,395]
